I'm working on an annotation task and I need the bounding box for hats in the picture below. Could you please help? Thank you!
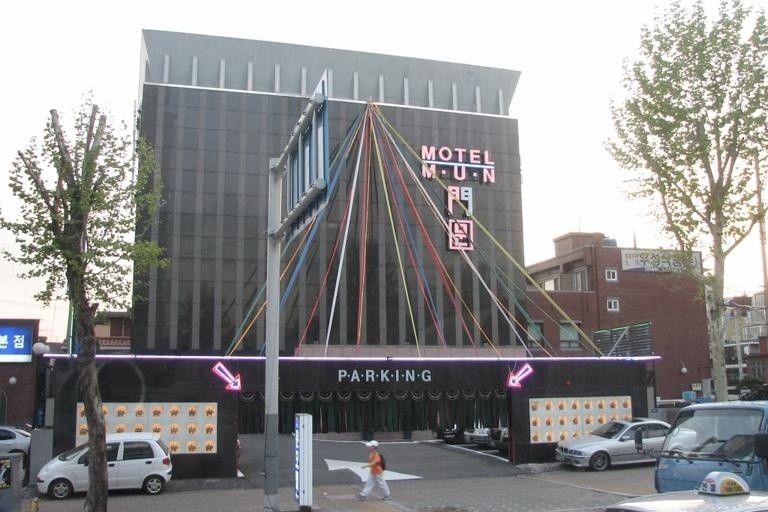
[366,440,378,447]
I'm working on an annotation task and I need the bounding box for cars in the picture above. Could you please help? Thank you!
[432,418,509,455]
[657,399,686,408]
[554,417,671,471]
[603,471,768,512]
[0,422,34,469]
[36,432,174,500]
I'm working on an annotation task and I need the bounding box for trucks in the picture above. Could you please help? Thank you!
[655,401,768,493]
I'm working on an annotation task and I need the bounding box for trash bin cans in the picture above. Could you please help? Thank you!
[403,424,411,439]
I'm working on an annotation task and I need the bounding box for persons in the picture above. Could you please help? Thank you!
[358,439,392,501]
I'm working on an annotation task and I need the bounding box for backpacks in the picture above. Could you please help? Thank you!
[379,453,386,470]
